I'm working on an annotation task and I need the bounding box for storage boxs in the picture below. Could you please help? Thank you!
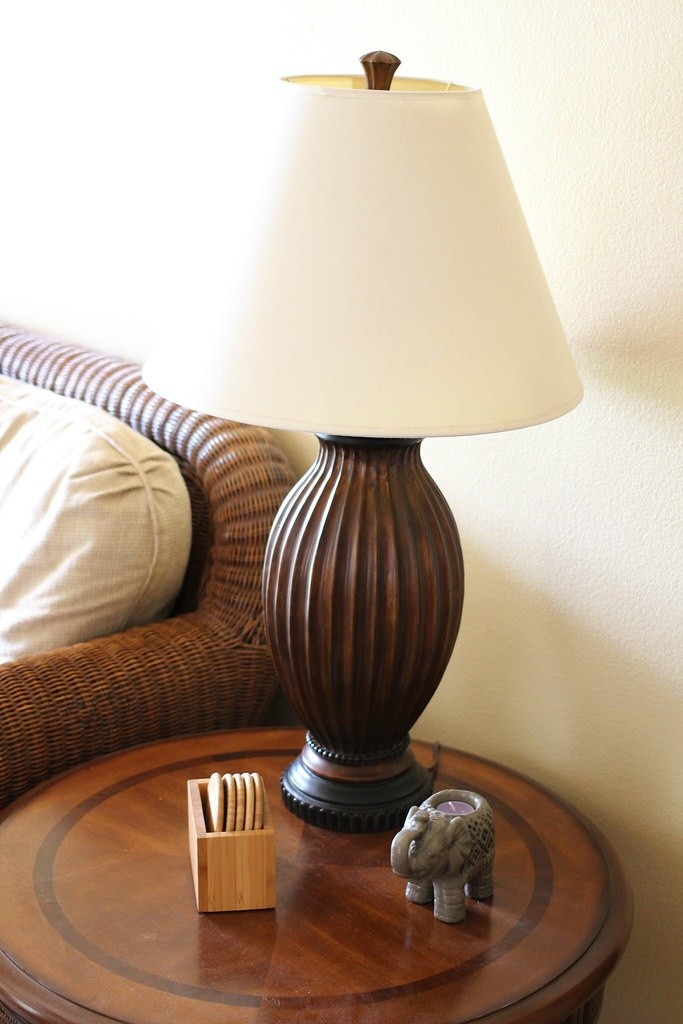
[185,778,279,913]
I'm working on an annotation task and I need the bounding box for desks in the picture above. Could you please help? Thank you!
[1,722,635,1023]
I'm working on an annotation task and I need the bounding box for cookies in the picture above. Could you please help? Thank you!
[203,772,264,832]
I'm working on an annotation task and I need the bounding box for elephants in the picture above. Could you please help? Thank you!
[388,784,496,925]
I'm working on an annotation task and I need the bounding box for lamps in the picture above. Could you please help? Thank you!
[141,49,581,827]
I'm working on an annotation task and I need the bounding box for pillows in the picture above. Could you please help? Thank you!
[1,374,192,663]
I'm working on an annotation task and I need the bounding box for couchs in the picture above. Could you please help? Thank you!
[1,306,293,805]
